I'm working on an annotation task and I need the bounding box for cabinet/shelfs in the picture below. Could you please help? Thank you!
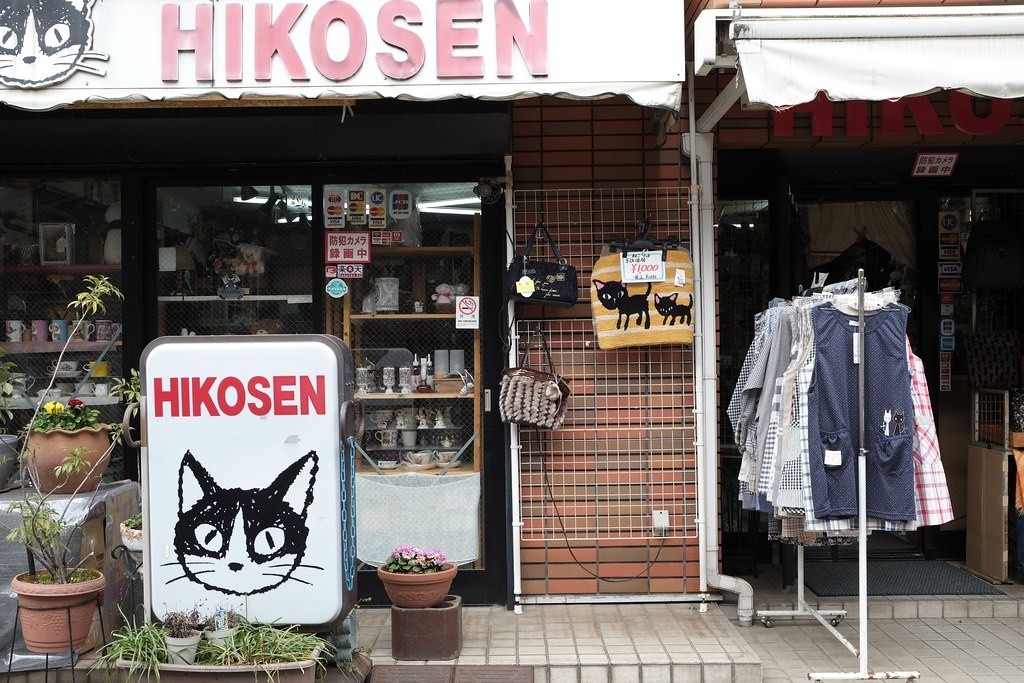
[343,212,485,572]
[0,264,124,410]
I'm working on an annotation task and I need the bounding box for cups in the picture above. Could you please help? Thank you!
[51,360,79,371]
[49,319,68,342]
[6,320,26,343]
[46,366,56,373]
[375,430,397,447]
[56,383,74,393]
[402,431,418,448]
[432,450,457,463]
[95,320,120,342]
[90,383,111,397]
[82,362,108,377]
[370,410,394,429]
[31,320,48,342]
[377,461,397,466]
[395,408,418,429]
[407,452,432,465]
[74,383,94,393]
[73,320,95,343]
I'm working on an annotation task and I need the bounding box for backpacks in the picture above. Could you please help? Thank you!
[964,211,1023,291]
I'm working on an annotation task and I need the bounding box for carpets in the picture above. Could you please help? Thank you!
[795,560,1006,597]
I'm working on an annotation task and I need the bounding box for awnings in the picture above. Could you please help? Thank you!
[695,15,1024,134]
[0,0,687,154]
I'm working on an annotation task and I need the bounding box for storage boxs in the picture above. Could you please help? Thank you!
[39,223,76,266]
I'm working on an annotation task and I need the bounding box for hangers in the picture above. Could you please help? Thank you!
[795,276,912,314]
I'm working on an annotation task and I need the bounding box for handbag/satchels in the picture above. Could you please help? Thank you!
[498,329,572,431]
[507,221,578,309]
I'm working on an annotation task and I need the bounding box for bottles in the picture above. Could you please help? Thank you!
[374,260,400,314]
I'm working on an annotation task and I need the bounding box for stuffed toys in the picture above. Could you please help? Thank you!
[248,318,285,335]
[230,243,265,276]
[217,274,244,300]
[431,284,453,304]
[453,283,471,296]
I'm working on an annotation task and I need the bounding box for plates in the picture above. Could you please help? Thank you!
[437,461,461,467]
[407,463,437,470]
[46,374,54,377]
[377,464,401,469]
[69,393,95,397]
[50,370,82,377]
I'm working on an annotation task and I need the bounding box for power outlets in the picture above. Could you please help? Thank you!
[652,510,670,537]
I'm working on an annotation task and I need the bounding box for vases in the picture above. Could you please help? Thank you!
[25,423,112,494]
[203,623,239,648]
[980,425,1024,448]
[163,629,202,665]
[377,562,458,608]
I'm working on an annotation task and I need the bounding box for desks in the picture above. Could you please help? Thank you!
[960,443,1024,585]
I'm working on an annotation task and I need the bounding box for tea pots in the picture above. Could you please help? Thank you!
[8,373,35,398]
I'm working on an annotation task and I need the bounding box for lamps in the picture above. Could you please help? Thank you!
[231,192,482,216]
[240,186,259,201]
[272,196,481,224]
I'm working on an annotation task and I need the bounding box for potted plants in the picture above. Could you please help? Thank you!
[85,599,338,683]
[0,274,140,652]
[0,347,26,490]
[120,512,144,551]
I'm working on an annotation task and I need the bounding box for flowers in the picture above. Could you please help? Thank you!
[22,400,102,432]
[1011,387,1024,433]
[385,544,448,573]
